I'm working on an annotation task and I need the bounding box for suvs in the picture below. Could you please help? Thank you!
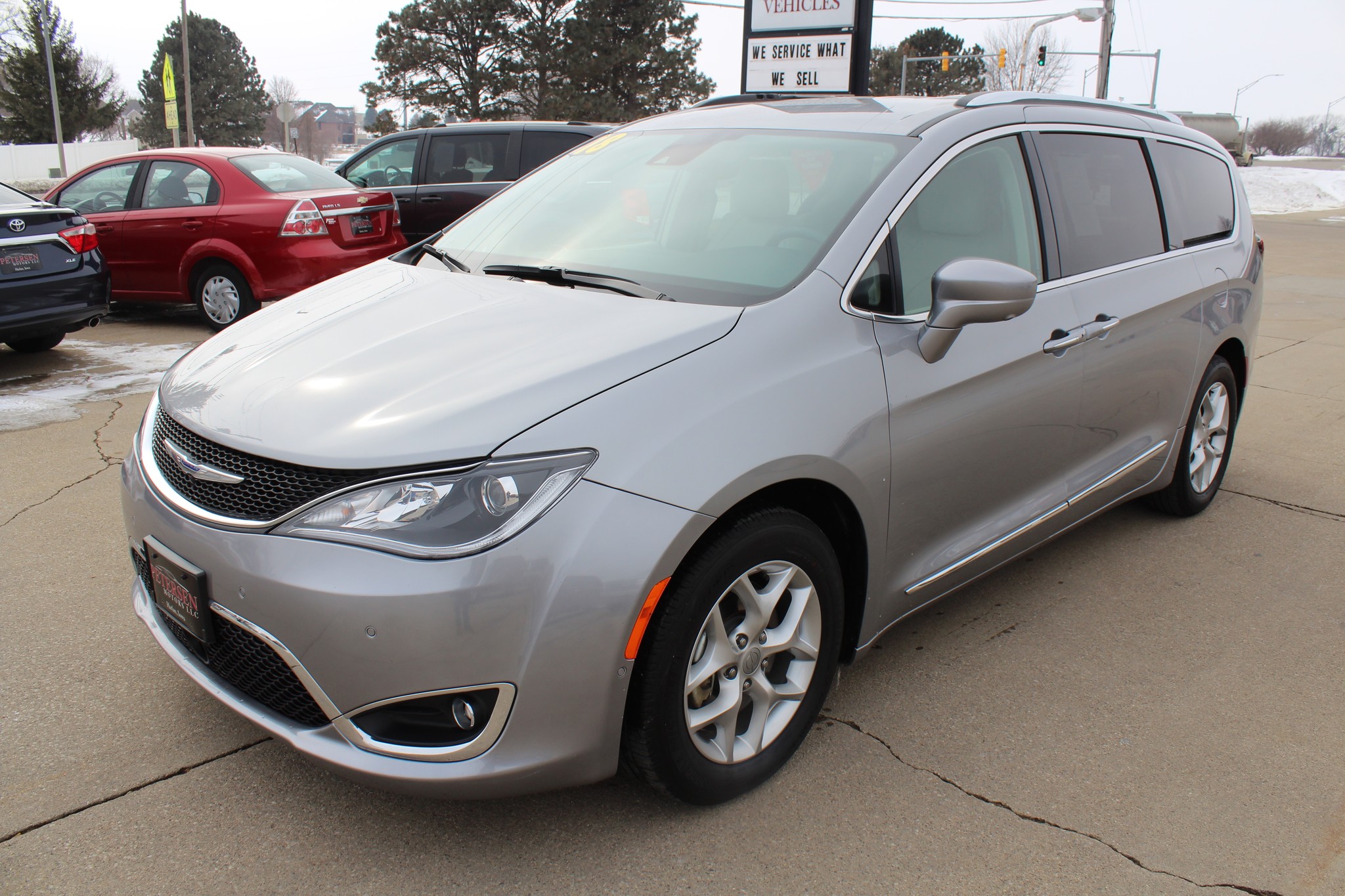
[114,91,1269,816]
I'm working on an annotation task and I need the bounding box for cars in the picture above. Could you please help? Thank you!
[37,144,407,336]
[324,120,618,250]
[1,178,109,364]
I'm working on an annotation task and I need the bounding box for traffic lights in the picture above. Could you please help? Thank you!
[1037,46,1046,65]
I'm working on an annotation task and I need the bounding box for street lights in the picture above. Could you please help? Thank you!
[1015,6,1107,92]
